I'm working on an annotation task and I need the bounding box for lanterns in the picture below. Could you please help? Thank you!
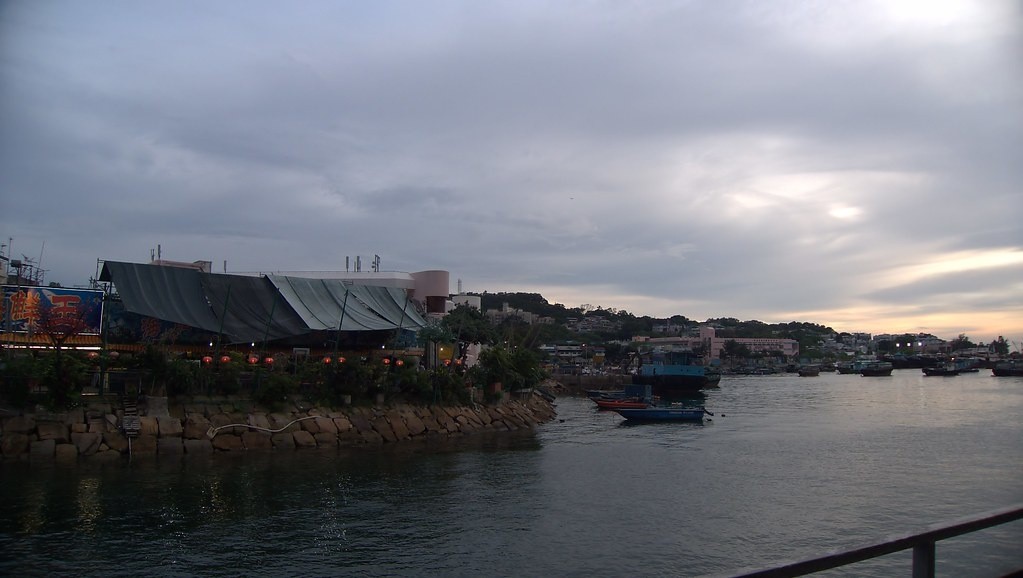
[395,360,403,366]
[337,357,346,362]
[203,356,213,363]
[323,357,331,363]
[249,357,258,363]
[383,359,390,364]
[109,351,119,359]
[221,356,231,363]
[264,358,274,363]
[455,360,462,364]
[88,352,98,359]
[445,359,451,364]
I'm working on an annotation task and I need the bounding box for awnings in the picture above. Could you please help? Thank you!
[99,261,234,337]
[345,284,430,332]
[198,273,312,344]
[266,275,399,331]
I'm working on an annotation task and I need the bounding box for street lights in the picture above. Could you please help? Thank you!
[382,344,389,359]
[210,334,220,395]
[323,340,338,365]
[251,338,263,396]
[583,343,587,366]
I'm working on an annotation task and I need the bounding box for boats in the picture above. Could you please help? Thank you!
[612,405,714,421]
[583,384,660,403]
[835,355,879,375]
[631,349,708,394]
[735,361,835,377]
[884,349,1023,378]
[597,399,647,411]
[590,395,647,404]
[858,360,893,377]
[697,358,723,389]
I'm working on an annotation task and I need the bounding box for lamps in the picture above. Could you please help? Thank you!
[382,344,385,349]
[209,337,213,347]
[252,342,255,346]
[440,346,443,350]
